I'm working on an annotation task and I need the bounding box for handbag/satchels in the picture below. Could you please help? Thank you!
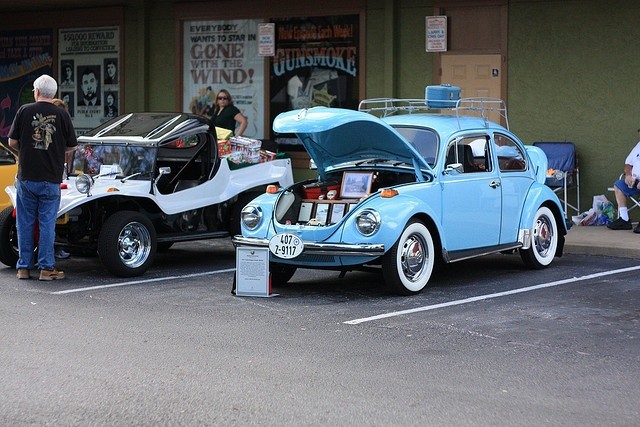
[597,202,617,225]
[592,195,608,213]
[572,208,597,226]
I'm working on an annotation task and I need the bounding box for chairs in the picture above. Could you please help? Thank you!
[532,140,582,220]
[449,144,476,172]
[607,172,640,220]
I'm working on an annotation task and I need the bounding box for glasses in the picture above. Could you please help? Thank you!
[218,96,227,100]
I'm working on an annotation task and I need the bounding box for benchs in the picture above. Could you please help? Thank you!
[473,156,525,171]
[135,156,202,180]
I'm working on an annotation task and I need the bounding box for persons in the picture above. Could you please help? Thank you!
[104,61,118,84]
[199,86,215,111]
[607,128,640,234]
[61,63,74,85]
[104,93,118,117]
[77,69,101,106]
[210,90,247,138]
[61,92,72,117]
[51,99,71,259]
[7,74,78,280]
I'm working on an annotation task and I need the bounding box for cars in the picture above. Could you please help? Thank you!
[231,84,567,295]
[0,111,295,277]
[0,142,83,227]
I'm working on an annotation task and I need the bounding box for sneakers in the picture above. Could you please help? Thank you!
[633,222,640,233]
[54,250,70,258]
[607,217,632,230]
[17,269,28,278]
[39,269,64,280]
